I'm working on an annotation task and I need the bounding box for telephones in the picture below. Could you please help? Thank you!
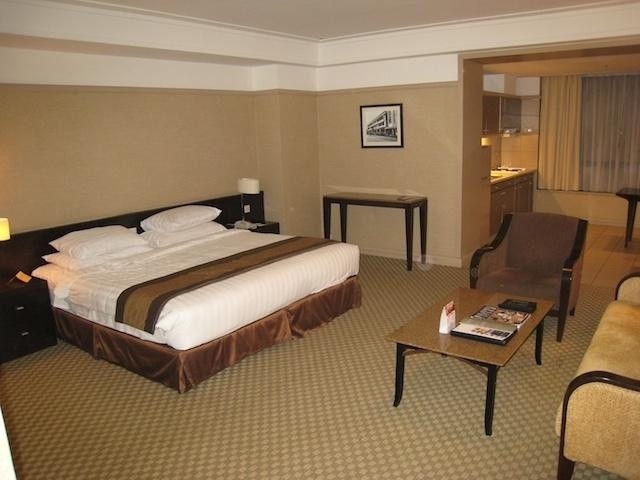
[234,221,253,229]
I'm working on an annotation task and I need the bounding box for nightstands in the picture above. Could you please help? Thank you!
[230,220,280,234]
[0,275,56,365]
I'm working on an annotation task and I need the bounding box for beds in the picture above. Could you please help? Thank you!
[27,189,362,394]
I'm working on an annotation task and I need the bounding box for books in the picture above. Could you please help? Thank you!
[452,299,537,346]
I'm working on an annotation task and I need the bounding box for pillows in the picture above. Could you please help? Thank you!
[140,205,223,233]
[48,223,149,261]
[139,221,227,246]
[39,242,152,270]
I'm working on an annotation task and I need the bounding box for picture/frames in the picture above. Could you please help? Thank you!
[359,104,404,148]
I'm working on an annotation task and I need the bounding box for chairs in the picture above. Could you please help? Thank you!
[467,210,589,344]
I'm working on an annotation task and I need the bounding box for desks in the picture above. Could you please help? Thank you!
[617,188,640,247]
[323,192,428,272]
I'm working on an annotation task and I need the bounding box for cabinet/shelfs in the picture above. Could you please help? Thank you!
[490,176,515,236]
[515,170,535,212]
[482,92,542,136]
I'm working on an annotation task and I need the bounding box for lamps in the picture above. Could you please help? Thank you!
[234,177,261,226]
[1,217,11,241]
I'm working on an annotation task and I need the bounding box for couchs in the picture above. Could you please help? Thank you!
[555,271,639,479]
